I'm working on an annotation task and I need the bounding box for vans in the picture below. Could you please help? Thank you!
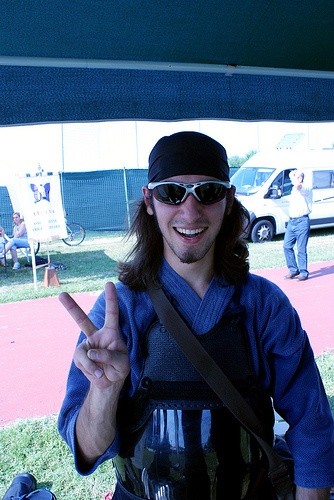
[228,148,334,243]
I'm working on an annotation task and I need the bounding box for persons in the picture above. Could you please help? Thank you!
[58,131,334,500]
[284,170,312,280]
[0,212,28,270]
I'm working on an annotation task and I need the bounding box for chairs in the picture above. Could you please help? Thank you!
[3,234,41,273]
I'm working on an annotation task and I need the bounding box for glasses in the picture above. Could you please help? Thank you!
[147,181,231,206]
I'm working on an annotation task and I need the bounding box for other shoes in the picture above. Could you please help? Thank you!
[293,274,308,280]
[12,261,21,270]
[284,273,296,278]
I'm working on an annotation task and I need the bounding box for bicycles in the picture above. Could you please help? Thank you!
[20,217,86,255]
